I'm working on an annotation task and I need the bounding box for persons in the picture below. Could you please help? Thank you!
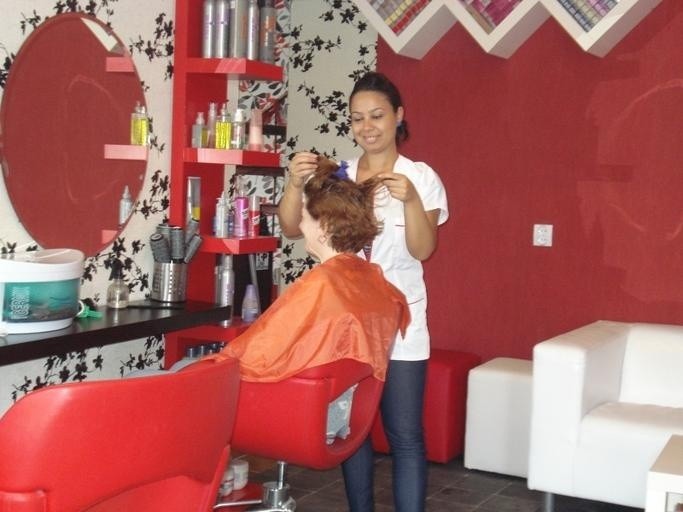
[123,154,412,497]
[277,72,451,512]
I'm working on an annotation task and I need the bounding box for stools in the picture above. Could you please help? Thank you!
[371,346,531,481]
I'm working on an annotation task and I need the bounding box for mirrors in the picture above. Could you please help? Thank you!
[0,14,149,262]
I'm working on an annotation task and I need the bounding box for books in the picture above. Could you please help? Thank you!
[369,0,621,37]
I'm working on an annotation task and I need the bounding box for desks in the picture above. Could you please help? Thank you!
[646,435,679,505]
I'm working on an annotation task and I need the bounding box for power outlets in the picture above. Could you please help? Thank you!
[533,224,553,247]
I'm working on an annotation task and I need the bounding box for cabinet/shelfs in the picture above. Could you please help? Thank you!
[164,2,285,369]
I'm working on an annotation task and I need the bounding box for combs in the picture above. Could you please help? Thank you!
[149,218,201,263]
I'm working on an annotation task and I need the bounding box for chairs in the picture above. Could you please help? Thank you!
[214,354,389,512]
[527,319,680,507]
[1,357,244,512]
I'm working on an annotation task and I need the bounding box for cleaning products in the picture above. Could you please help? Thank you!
[106,257,129,309]
